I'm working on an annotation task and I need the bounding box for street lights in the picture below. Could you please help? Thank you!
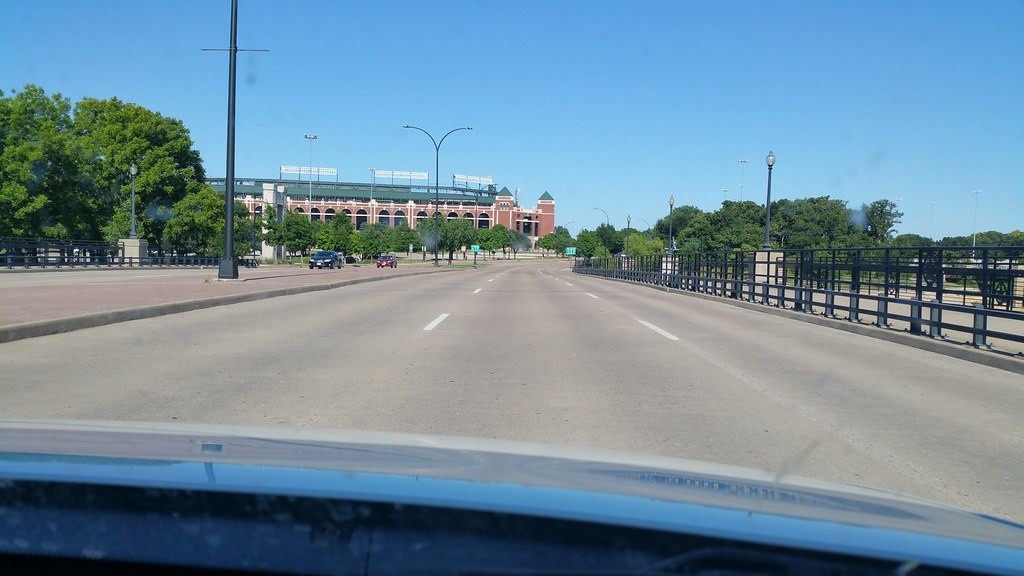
[761,150,777,252]
[594,207,610,227]
[562,222,575,239]
[971,188,981,263]
[737,159,750,201]
[402,124,474,267]
[303,133,318,224]
[667,195,676,253]
[722,188,730,201]
[626,214,632,255]
[455,183,500,264]
[128,163,138,239]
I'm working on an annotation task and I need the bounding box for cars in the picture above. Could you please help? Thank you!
[308,250,343,270]
[377,255,397,268]
